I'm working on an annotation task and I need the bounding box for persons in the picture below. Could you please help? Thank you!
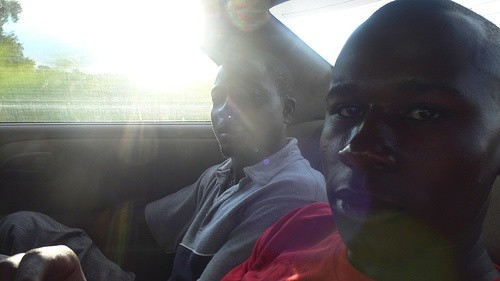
[1,48,330,281]
[0,0,500,281]
